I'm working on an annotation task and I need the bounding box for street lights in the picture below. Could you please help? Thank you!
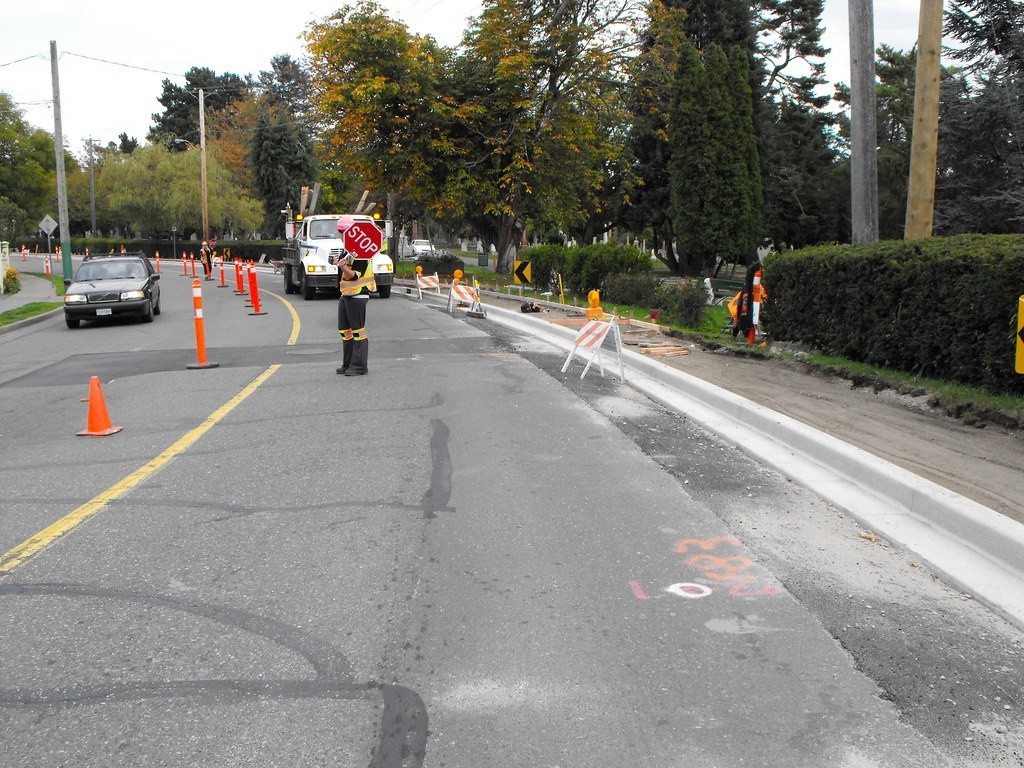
[174,138,208,241]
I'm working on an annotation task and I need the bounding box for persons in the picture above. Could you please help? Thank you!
[200,241,213,281]
[336,215,377,376]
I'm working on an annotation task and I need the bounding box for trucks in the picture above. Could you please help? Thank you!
[281,201,393,302]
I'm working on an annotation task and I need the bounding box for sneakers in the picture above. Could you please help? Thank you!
[337,368,347,373]
[345,369,368,376]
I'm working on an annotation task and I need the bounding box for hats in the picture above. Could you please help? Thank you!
[336,216,354,230]
[202,241,208,246]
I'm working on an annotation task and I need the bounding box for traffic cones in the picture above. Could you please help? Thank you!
[75,375,124,436]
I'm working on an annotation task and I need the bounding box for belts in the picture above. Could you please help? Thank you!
[351,295,369,298]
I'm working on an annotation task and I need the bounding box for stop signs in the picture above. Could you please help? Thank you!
[209,238,217,248]
[343,221,382,261]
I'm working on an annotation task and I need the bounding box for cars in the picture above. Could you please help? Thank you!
[62,253,161,329]
[410,240,436,256]
